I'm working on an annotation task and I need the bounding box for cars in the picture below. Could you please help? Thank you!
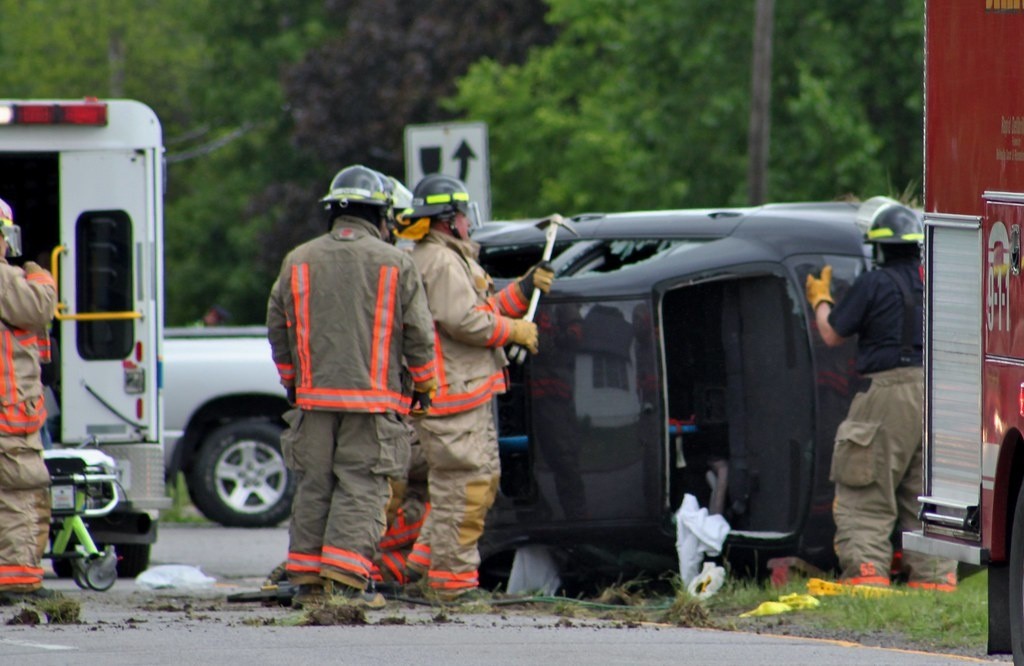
[401,194,925,594]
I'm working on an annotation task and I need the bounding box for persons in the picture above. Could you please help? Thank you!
[267,165,555,611]
[805,206,959,593]
[0,198,56,607]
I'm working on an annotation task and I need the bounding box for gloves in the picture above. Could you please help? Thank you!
[24,260,44,277]
[804,266,834,312]
[409,377,438,417]
[505,315,539,357]
[515,261,554,306]
[281,379,297,407]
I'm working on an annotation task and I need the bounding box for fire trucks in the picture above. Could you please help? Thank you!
[921,0,1024,666]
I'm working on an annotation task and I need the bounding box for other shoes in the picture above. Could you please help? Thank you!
[323,578,386,609]
[296,584,334,608]
[402,563,426,599]
[0,586,63,606]
[421,583,502,609]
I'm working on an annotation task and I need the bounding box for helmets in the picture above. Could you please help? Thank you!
[0,198,22,257]
[317,163,387,204]
[375,170,397,205]
[401,174,469,219]
[863,204,925,243]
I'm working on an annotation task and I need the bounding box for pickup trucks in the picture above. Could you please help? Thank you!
[159,316,293,530]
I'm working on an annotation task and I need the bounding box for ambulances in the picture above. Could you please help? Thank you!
[0,93,185,584]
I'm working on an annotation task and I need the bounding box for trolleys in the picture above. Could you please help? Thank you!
[40,444,123,593]
